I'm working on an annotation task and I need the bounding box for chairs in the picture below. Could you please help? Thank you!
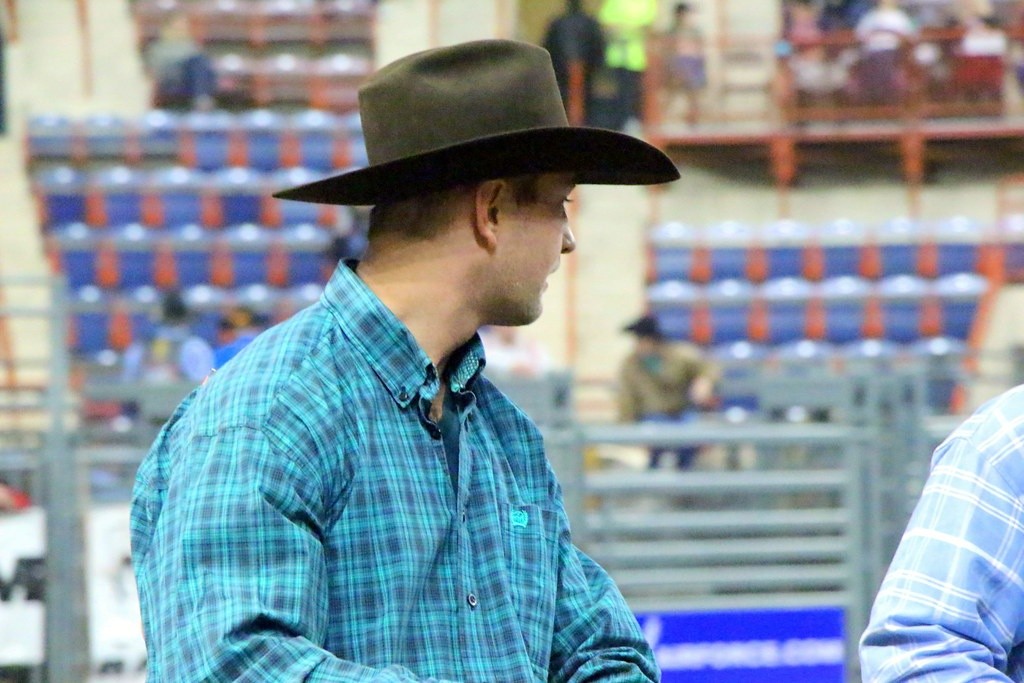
[26,0,372,424]
[648,223,1024,424]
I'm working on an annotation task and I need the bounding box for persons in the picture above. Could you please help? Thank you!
[117,296,275,444]
[813,1,1007,114]
[544,0,705,128]
[125,40,661,682]
[614,317,721,469]
[857,386,1024,683]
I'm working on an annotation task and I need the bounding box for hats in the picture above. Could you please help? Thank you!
[270,40,683,206]
[626,316,663,339]
